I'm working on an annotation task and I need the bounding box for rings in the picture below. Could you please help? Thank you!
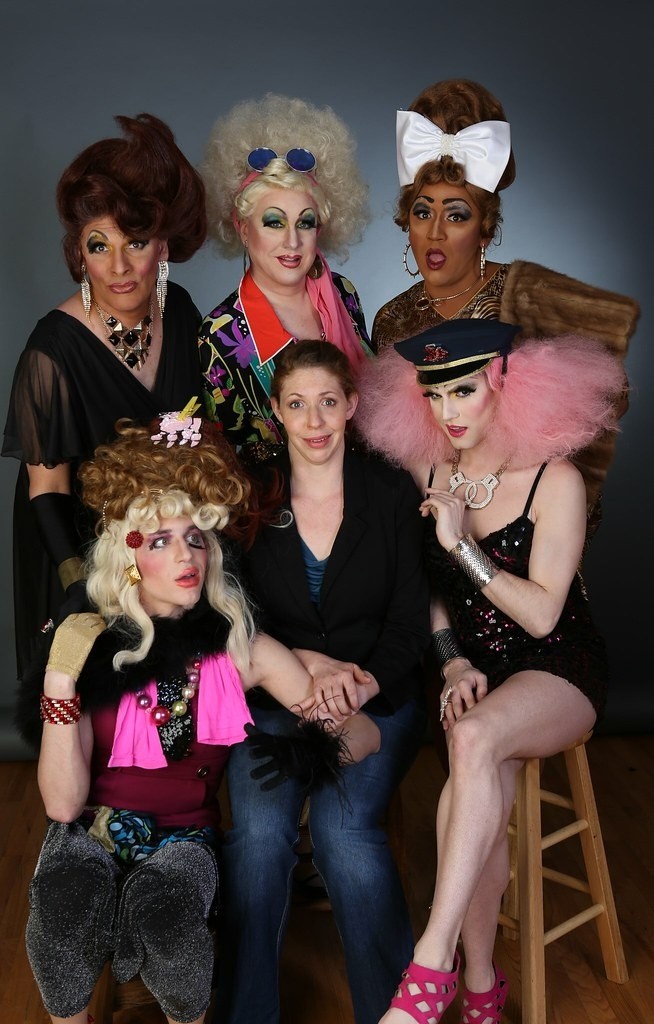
[440,687,452,721]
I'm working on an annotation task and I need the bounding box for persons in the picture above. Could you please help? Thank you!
[218,339,431,1024]
[373,81,639,525]
[0,114,206,682]
[199,94,372,470]
[24,395,382,1024]
[377,321,606,1024]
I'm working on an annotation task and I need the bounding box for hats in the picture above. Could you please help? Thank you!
[392,318,525,388]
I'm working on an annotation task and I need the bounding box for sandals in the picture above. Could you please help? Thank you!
[459,959,510,1024]
[380,949,461,1024]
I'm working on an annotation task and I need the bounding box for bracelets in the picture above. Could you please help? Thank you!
[432,628,463,677]
[448,533,500,590]
[39,696,82,725]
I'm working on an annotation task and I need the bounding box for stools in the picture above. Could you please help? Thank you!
[89,730,629,1024]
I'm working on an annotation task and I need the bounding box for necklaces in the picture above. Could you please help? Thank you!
[93,300,155,369]
[414,272,480,310]
[449,449,512,510]
[136,652,201,726]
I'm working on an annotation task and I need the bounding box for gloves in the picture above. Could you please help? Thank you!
[243,720,356,818]
[45,611,107,682]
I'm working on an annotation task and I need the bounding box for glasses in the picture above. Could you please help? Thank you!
[246,146,317,173]
